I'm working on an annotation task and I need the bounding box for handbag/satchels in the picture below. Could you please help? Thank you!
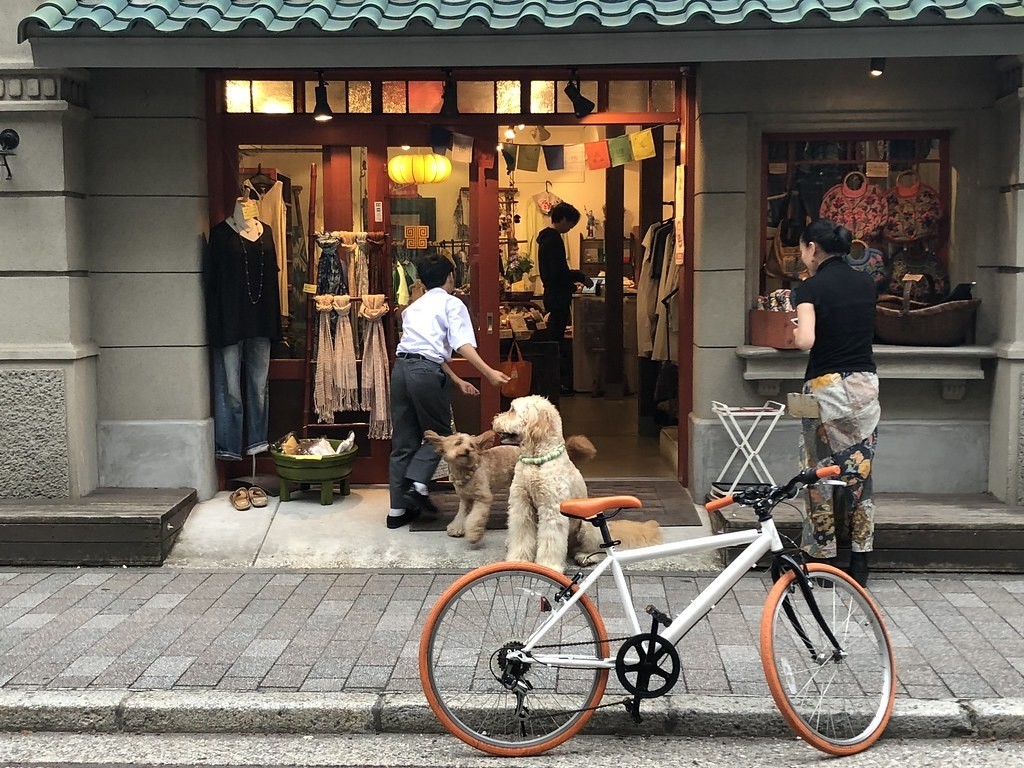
[879,170,944,242]
[815,171,888,239]
[500,341,532,397]
[884,239,949,302]
[764,219,810,281]
[841,239,884,282]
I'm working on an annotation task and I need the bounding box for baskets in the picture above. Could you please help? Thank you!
[873,282,982,347]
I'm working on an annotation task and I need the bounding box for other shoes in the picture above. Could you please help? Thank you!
[807,557,835,588]
[386,509,417,529]
[560,385,575,397]
[400,483,439,513]
[848,560,870,588]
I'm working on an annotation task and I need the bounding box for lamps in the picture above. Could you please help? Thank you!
[505,125,515,139]
[538,126,550,141]
[564,69,595,118]
[870,58,885,76]
[440,71,459,116]
[311,70,333,121]
[388,147,452,185]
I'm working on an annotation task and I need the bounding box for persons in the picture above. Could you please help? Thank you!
[756,286,796,313]
[792,218,883,588]
[535,203,595,398]
[206,194,280,463]
[387,252,512,528]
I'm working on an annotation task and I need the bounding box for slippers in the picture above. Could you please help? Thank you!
[229,487,251,510]
[248,487,269,507]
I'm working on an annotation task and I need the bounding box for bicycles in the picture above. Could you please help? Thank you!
[417,457,900,757]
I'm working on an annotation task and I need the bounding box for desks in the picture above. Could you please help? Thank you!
[572,295,639,393]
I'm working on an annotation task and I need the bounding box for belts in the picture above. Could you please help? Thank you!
[397,353,426,360]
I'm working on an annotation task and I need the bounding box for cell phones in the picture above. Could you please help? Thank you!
[791,318,798,326]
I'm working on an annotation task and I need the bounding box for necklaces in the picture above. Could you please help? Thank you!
[231,212,264,304]
[233,217,255,234]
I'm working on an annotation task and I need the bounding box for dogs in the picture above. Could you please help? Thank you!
[423,395,660,576]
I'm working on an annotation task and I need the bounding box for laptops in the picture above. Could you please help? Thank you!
[574,277,606,296]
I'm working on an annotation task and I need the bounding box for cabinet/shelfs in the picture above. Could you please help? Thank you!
[710,399,786,499]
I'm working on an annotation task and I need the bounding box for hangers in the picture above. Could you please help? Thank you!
[661,202,675,241]
[246,162,276,187]
[394,239,456,260]
[544,181,553,194]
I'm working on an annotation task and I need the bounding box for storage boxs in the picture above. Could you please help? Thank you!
[749,310,799,349]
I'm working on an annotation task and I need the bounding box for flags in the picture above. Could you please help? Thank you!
[503,141,519,171]
[429,121,454,156]
[584,140,613,169]
[608,134,636,167]
[564,143,586,172]
[629,129,657,161]
[542,144,565,172]
[452,131,473,163]
[516,143,540,172]
